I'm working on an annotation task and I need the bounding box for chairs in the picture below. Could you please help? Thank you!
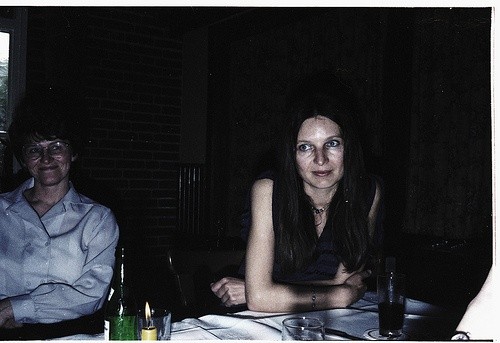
[165,249,235,304]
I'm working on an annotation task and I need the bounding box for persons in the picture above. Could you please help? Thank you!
[0,96,120,340]
[209,101,382,312]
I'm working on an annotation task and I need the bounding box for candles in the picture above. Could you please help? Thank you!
[140,300,158,340]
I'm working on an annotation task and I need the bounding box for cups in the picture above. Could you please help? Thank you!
[283,316,327,340]
[137,307,172,340]
[376,273,407,338]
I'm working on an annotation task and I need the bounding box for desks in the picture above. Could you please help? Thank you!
[0,290,464,341]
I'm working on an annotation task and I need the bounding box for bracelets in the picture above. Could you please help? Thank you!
[308,287,317,311]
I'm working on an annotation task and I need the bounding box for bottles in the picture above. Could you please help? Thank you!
[102,247,139,340]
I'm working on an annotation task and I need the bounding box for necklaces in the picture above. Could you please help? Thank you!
[308,202,332,214]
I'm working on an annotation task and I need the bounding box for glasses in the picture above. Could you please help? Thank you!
[22,140,72,160]
